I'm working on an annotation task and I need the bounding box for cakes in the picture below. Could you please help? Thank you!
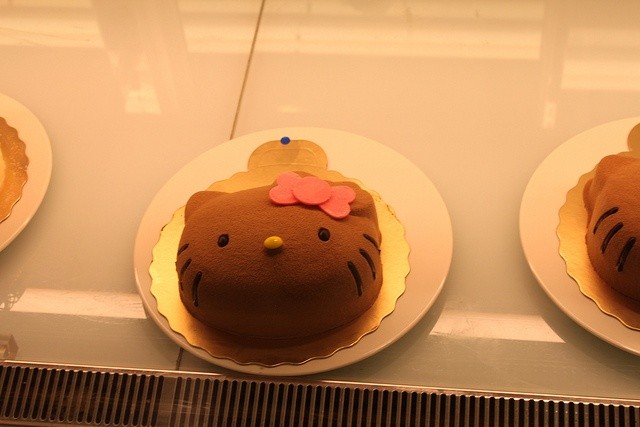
[174,171,384,343]
[582,123,639,304]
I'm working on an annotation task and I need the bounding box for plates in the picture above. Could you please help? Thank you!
[0,92,56,251]
[133,125,456,377]
[518,117,640,357]
